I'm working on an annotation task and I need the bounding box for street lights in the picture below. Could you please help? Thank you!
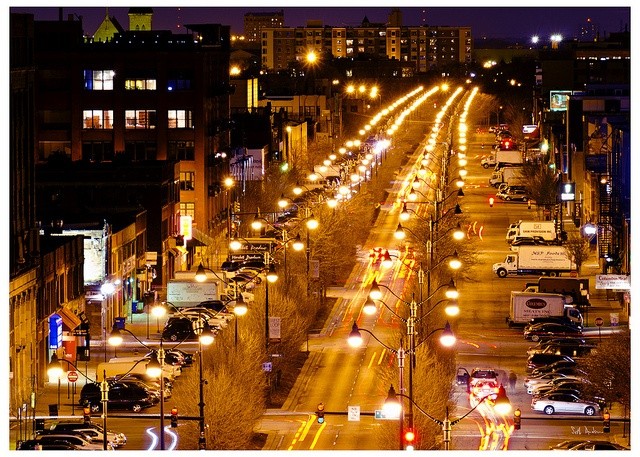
[158,299,247,450]
[109,326,217,446]
[45,358,163,449]
[347,321,456,428]
[228,219,322,300]
[394,223,466,306]
[362,278,467,370]
[223,177,235,237]
[399,201,462,263]
[380,251,464,315]
[274,87,480,228]
[99,281,114,361]
[266,267,279,345]
[193,266,280,347]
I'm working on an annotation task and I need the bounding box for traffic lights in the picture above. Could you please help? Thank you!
[399,430,418,450]
[513,407,522,429]
[602,411,614,431]
[170,407,179,427]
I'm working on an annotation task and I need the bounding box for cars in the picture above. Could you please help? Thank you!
[524,324,594,394]
[489,125,513,148]
[139,349,193,372]
[227,266,264,296]
[18,420,129,449]
[496,187,530,201]
[161,300,234,339]
[454,367,502,397]
[524,321,585,339]
[113,372,170,399]
[529,393,602,416]
[553,438,628,450]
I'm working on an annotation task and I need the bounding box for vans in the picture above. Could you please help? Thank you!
[79,380,150,412]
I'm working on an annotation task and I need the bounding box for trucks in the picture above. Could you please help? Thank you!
[525,276,589,310]
[493,247,578,277]
[506,220,561,245]
[490,166,535,187]
[508,290,583,325]
[226,237,285,262]
[167,279,243,305]
[98,362,173,385]
[479,149,525,169]
